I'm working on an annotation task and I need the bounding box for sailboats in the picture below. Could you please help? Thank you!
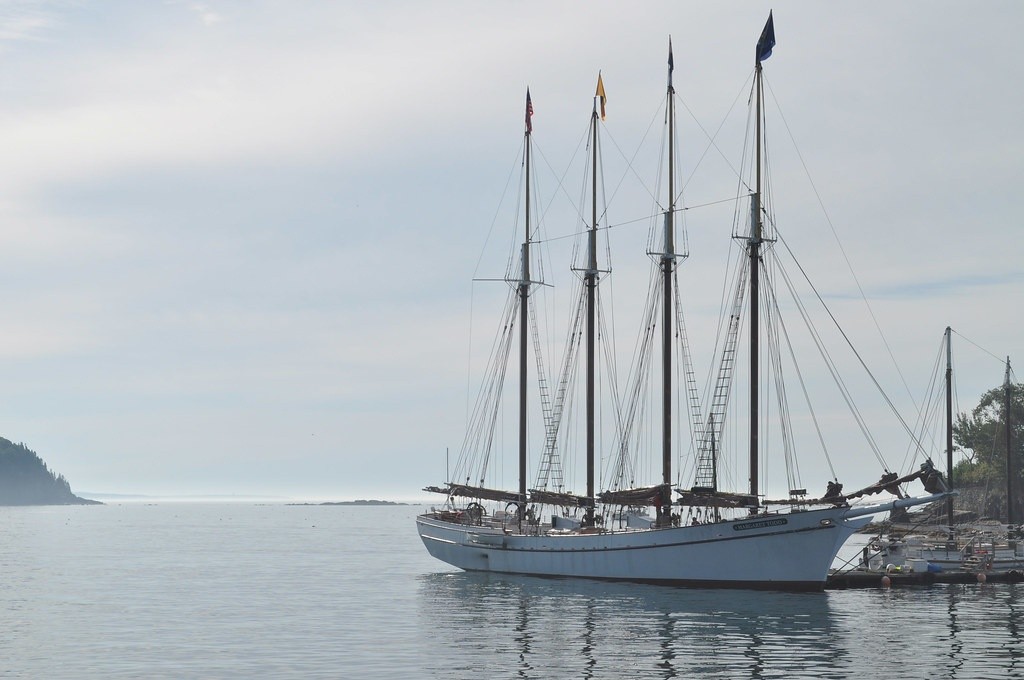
[414,10,956,586]
[854,326,1024,581]
